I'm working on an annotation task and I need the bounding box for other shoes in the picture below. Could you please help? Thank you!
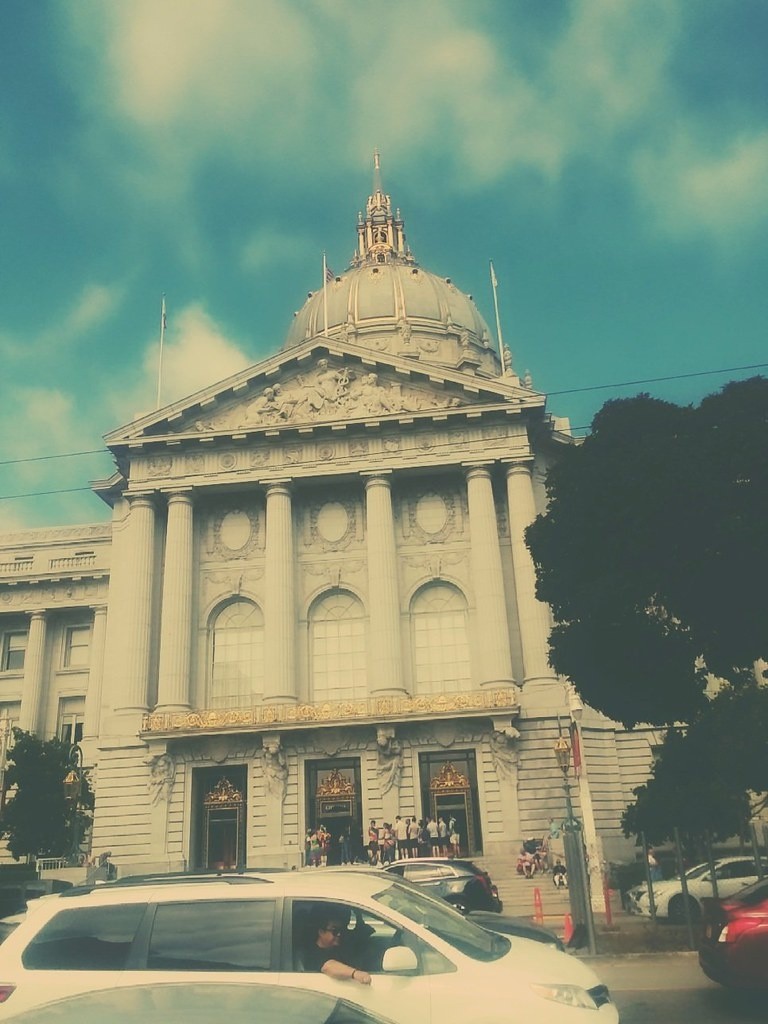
[377,862,382,866]
[318,862,327,867]
[341,862,351,866]
[526,875,533,879]
[384,861,389,866]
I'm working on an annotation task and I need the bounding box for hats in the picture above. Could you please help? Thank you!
[306,829,315,837]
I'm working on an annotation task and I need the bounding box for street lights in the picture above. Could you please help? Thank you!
[554,714,581,833]
[62,745,84,867]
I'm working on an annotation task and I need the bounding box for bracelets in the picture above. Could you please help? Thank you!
[352,969,363,979]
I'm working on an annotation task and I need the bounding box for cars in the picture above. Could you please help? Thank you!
[0,979,394,1024]
[697,877,768,993]
[628,855,768,923]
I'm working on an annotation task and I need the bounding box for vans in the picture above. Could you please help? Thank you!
[0,867,621,1023]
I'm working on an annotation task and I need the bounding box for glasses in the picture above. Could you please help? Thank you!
[557,862,561,864]
[322,927,345,936]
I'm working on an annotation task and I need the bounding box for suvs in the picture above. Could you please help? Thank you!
[379,857,504,914]
[307,865,566,952]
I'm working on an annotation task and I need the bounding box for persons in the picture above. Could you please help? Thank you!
[643,845,689,881]
[516,817,568,889]
[368,812,460,866]
[298,901,376,986]
[305,824,363,867]
